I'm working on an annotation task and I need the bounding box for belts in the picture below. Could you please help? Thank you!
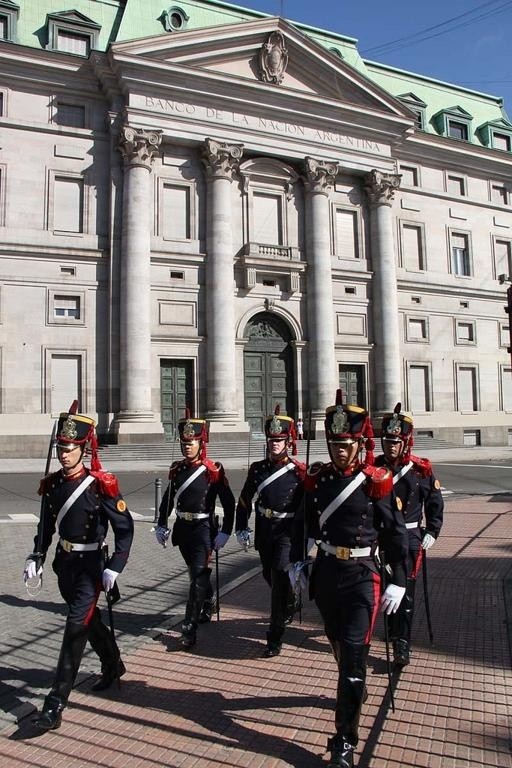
[257,507,293,519]
[59,537,106,551]
[320,543,371,560]
[380,521,418,529]
[176,513,210,518]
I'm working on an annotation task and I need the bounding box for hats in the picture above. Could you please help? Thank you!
[264,403,298,456]
[324,389,375,464]
[379,401,415,463]
[57,399,102,473]
[176,407,209,459]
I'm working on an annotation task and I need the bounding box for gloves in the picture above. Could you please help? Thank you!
[155,526,171,546]
[422,534,435,550]
[236,530,250,552]
[102,569,119,591]
[380,584,407,615]
[290,566,308,595]
[214,532,229,551]
[24,558,43,579]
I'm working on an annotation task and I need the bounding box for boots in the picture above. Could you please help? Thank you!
[391,580,412,665]
[328,642,370,768]
[167,566,212,651]
[262,570,302,624]
[29,620,88,729]
[266,570,288,658]
[200,581,219,625]
[87,607,126,690]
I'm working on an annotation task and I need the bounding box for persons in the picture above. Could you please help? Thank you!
[295,406,408,767]
[374,412,444,668]
[156,416,236,648]
[231,414,310,658]
[295,416,305,440]
[24,412,135,732]
